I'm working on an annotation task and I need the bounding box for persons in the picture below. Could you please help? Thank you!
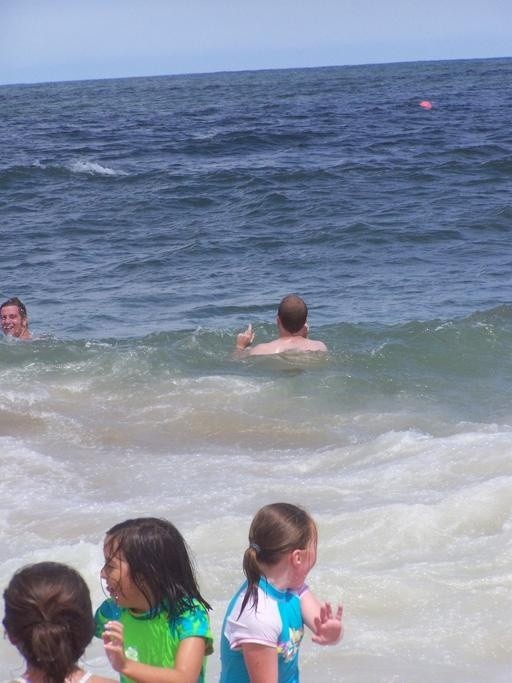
[218,502,343,682]
[93,518,214,683]
[236,296,326,355]
[1,561,123,683]
[0,298,33,340]
